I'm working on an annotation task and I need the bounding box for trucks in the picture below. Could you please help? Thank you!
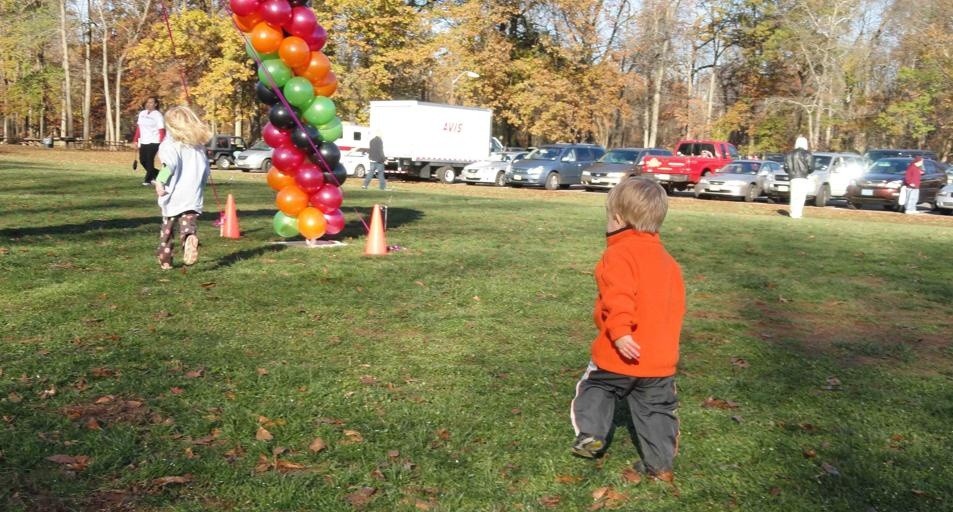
[369,99,503,185]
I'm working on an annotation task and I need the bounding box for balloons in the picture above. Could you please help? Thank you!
[224,1,346,239]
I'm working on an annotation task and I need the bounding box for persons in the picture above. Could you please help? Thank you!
[902,155,925,215]
[570,176,686,482]
[785,136,815,218]
[133,96,166,188]
[361,130,389,191]
[154,106,211,270]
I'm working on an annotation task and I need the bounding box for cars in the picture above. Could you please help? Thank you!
[506,143,606,190]
[341,146,369,178]
[580,147,673,192]
[844,157,948,212]
[458,150,530,185]
[769,155,785,169]
[694,160,787,202]
[935,180,953,212]
[0,131,131,148]
[234,139,275,173]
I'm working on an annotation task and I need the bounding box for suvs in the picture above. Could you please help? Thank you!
[768,152,869,207]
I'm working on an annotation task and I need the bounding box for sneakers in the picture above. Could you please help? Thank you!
[183,235,199,265]
[572,434,606,458]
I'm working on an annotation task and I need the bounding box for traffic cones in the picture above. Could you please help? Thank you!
[360,201,389,256]
[219,192,241,237]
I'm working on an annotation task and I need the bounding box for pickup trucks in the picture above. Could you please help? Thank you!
[640,140,739,196]
[204,135,247,170]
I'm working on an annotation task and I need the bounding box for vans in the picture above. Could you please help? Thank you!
[866,150,938,163]
[330,124,368,162]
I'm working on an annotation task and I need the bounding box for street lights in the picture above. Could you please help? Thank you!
[449,69,480,105]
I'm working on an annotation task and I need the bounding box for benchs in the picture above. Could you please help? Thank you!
[1,135,128,151]
